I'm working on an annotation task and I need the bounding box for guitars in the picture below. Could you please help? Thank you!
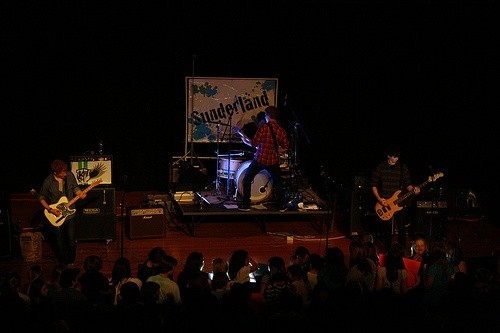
[233,126,288,169]
[43,179,102,227]
[374,171,444,221]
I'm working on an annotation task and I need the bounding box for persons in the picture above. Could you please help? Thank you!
[237,107,290,212]
[1,232,500,333]
[36,158,87,268]
[240,111,265,153]
[371,148,420,249]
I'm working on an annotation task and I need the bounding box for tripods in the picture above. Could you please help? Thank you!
[205,108,237,207]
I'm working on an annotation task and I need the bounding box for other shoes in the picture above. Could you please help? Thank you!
[238,205,251,211]
[279,206,288,212]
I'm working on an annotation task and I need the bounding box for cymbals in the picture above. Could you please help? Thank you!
[210,121,231,127]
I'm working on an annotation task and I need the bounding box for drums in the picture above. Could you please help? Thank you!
[217,157,246,179]
[234,160,275,204]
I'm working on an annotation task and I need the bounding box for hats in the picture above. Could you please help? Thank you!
[59,267,80,287]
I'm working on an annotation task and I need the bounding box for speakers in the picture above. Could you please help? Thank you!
[69,155,113,188]
[351,191,392,238]
[126,206,166,240]
[0,208,12,258]
[73,188,116,244]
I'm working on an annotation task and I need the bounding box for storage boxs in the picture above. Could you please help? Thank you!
[10,193,55,231]
[126,205,166,240]
[75,186,117,245]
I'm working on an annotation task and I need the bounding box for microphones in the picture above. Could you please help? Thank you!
[235,96,238,109]
[284,94,287,106]
